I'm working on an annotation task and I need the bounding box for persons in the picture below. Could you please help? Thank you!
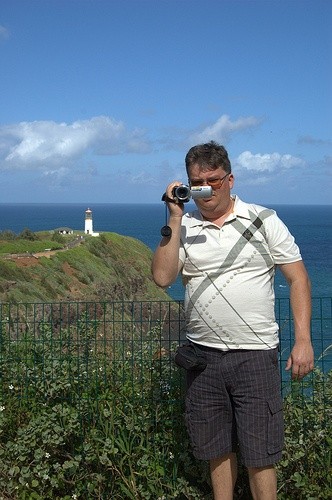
[151,141,314,500]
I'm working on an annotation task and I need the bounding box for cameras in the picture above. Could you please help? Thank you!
[173,184,213,200]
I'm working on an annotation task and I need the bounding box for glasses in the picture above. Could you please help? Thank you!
[189,172,231,187]
